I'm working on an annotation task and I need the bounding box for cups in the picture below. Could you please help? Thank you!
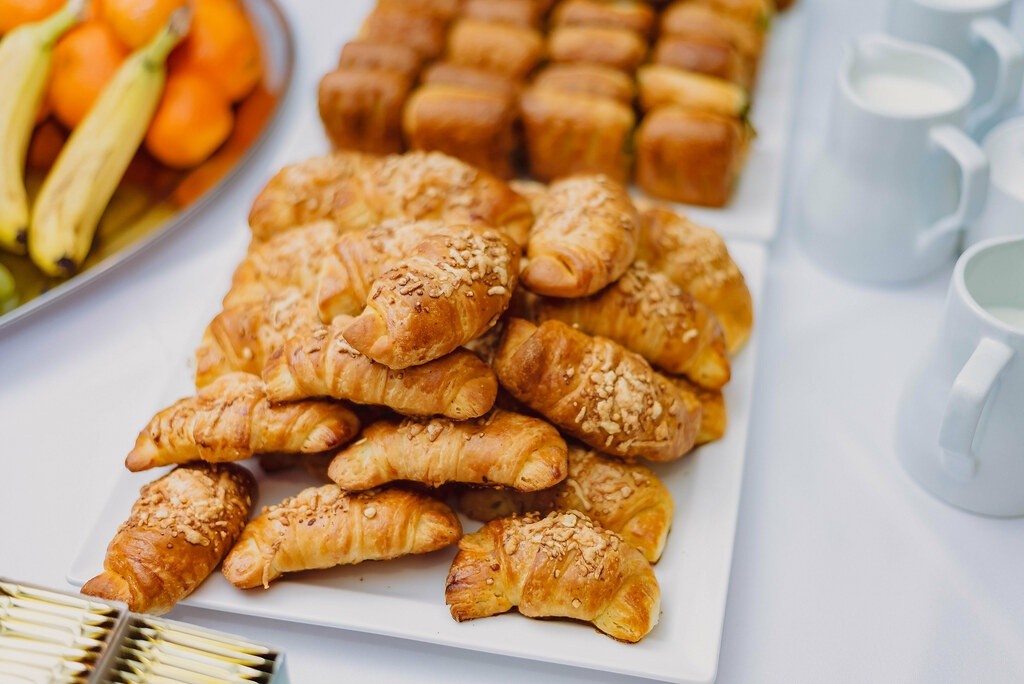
[888,231,1024,519]
[958,116,1024,260]
[882,0,1024,122]
[790,41,991,290]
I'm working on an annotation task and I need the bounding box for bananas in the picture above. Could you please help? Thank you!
[0,0,91,252]
[26,12,191,278]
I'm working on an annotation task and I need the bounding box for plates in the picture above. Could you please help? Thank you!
[67,239,767,684]
[279,0,805,241]
[0,0,296,332]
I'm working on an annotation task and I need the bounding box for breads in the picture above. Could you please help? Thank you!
[316,0,795,206]
[82,148,755,642]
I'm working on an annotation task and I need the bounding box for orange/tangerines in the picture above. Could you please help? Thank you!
[0,0,263,166]
